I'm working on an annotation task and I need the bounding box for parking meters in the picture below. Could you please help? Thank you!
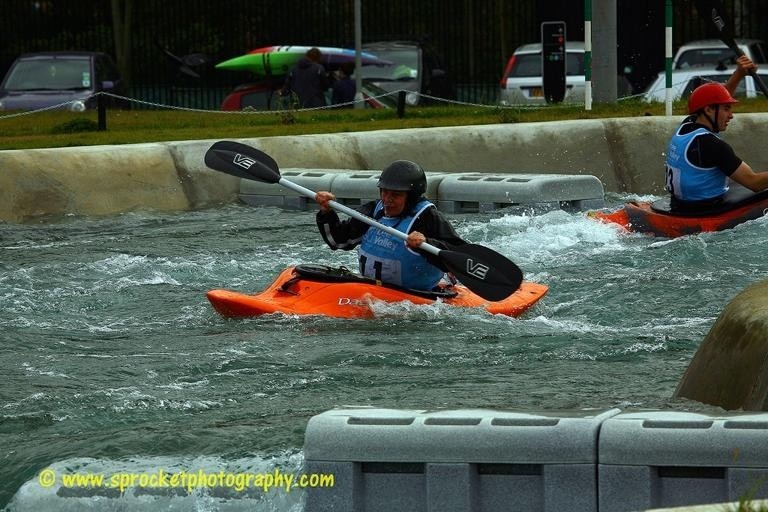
[540,21,566,105]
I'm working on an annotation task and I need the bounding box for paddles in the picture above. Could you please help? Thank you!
[205,140,522,302]
[694,0,767,97]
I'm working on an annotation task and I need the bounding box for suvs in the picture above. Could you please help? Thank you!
[500,42,634,106]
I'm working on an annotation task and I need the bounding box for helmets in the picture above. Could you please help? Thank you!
[688,82,738,112]
[377,160,427,196]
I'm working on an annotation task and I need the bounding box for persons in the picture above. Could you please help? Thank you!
[286,47,332,109]
[331,63,357,109]
[664,55,768,217]
[316,160,459,291]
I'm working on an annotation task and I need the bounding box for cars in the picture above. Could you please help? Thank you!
[221,81,404,111]
[641,40,767,104]
[0,52,131,114]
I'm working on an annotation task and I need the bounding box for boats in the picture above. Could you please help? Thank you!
[205,264,550,321]
[588,185,767,237]
[215,45,394,78]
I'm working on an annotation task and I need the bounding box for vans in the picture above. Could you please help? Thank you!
[362,41,422,105]
